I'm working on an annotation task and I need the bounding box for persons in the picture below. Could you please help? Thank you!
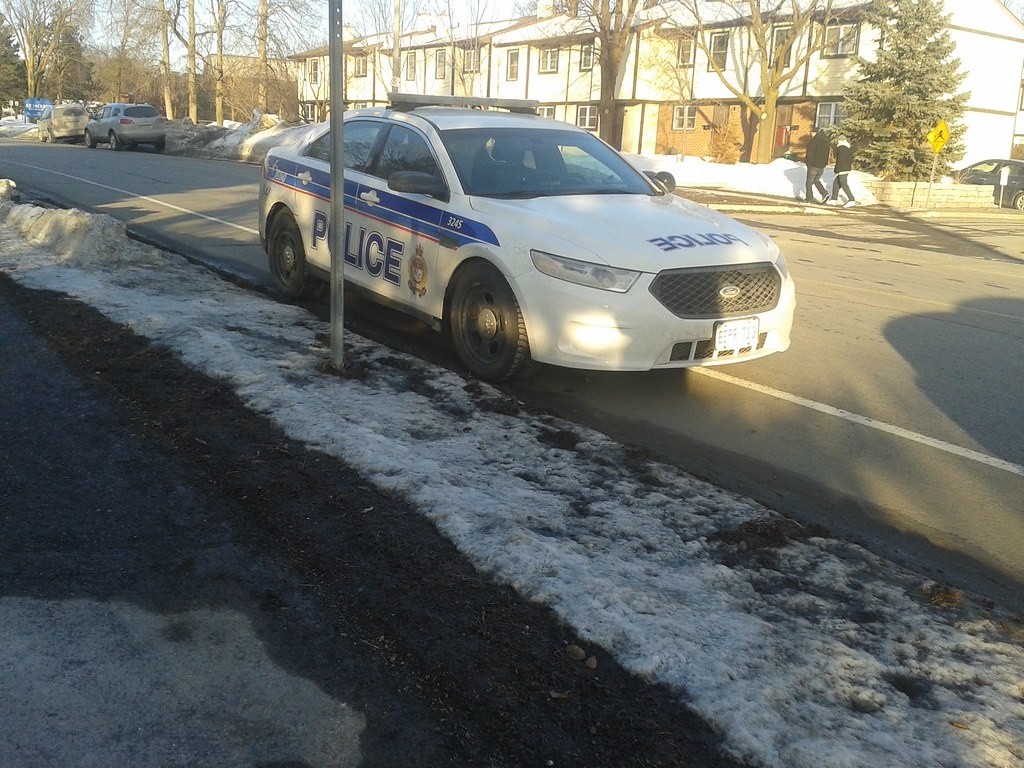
[826,134,856,208]
[803,126,830,205]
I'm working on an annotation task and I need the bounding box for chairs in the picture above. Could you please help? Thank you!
[385,126,437,177]
[485,137,531,194]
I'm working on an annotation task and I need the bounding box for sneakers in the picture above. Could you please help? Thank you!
[826,200,837,205]
[843,201,856,207]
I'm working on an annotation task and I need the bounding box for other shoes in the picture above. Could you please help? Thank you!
[820,194,829,205]
[802,198,815,203]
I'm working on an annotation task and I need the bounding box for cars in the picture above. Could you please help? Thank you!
[83,101,167,151]
[948,158,1024,211]
[32,103,90,142]
[259,91,799,388]
